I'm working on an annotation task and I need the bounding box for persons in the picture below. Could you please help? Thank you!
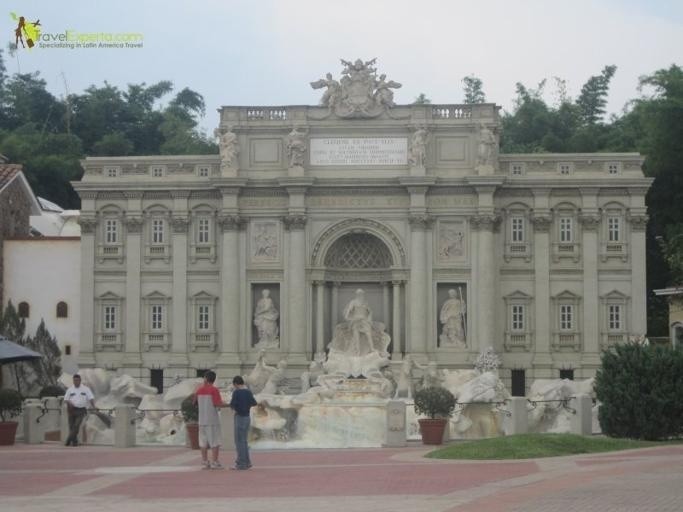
[440,289,467,349]
[229,375,257,469]
[259,349,287,395]
[64,374,98,446]
[254,289,280,348]
[310,58,402,107]
[343,288,374,354]
[192,371,231,469]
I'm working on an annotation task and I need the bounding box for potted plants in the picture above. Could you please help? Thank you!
[0,389,25,445]
[414,387,457,444]
[181,394,198,449]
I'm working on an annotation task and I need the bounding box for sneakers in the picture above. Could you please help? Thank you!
[200,461,210,469]
[210,463,224,469]
